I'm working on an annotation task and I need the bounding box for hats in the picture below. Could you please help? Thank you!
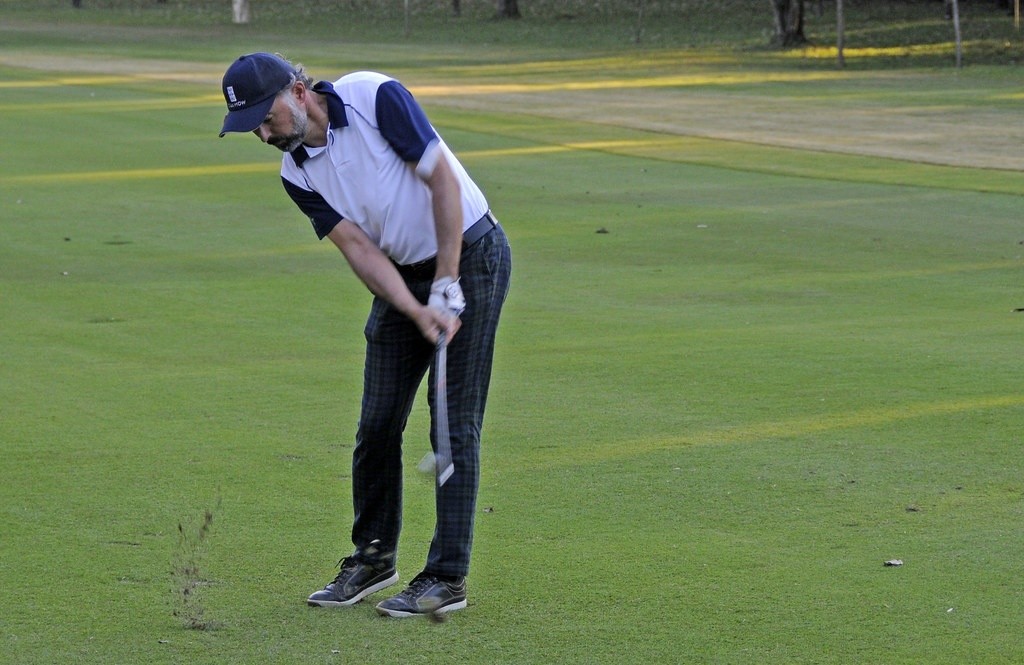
[218,53,307,135]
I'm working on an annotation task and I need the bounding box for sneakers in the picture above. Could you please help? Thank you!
[306,549,403,607]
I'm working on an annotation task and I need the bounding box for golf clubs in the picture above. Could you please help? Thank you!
[418,308,457,483]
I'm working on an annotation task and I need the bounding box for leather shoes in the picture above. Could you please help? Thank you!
[376,572,479,617]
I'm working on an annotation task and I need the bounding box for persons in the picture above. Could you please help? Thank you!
[218,51,511,618]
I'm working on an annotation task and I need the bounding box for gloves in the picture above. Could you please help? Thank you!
[429,276,466,317]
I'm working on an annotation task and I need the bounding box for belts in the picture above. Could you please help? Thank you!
[462,209,498,247]
